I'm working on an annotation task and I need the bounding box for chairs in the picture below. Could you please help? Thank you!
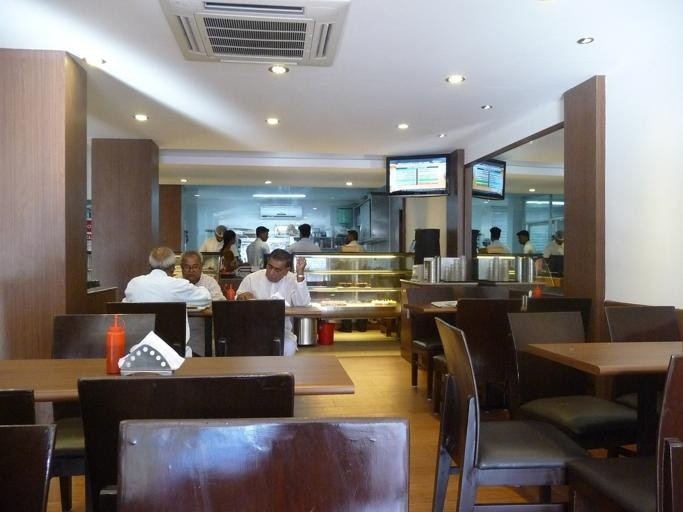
[605,305,678,416]
[49,276,287,356]
[566,354,681,510]
[432,315,593,510]
[405,287,594,415]
[505,310,641,505]
[1,371,410,510]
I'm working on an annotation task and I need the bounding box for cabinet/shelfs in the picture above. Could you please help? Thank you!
[292,251,412,314]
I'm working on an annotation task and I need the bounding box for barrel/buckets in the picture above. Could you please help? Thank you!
[515,255,536,283]
[317,323,336,345]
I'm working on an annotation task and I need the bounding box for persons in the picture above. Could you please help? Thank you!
[197,225,238,256]
[245,226,270,273]
[171,251,230,301]
[486,227,511,253]
[287,223,321,253]
[328,229,368,333]
[515,230,536,254]
[542,230,564,259]
[218,230,238,279]
[233,248,311,356]
[120,246,212,357]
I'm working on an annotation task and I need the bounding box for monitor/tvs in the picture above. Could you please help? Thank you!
[473,159,506,200]
[386,154,449,198]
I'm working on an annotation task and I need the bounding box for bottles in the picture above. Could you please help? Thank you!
[105,314,125,376]
[223,283,235,300]
[533,284,542,298]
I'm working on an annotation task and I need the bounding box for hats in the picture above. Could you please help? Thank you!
[256,227,268,232]
[299,224,310,230]
[490,228,500,233]
[516,230,527,235]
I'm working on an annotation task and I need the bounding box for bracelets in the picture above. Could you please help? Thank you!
[296,274,304,278]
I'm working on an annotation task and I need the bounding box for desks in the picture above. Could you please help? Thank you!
[525,340,682,455]
[1,352,357,402]
